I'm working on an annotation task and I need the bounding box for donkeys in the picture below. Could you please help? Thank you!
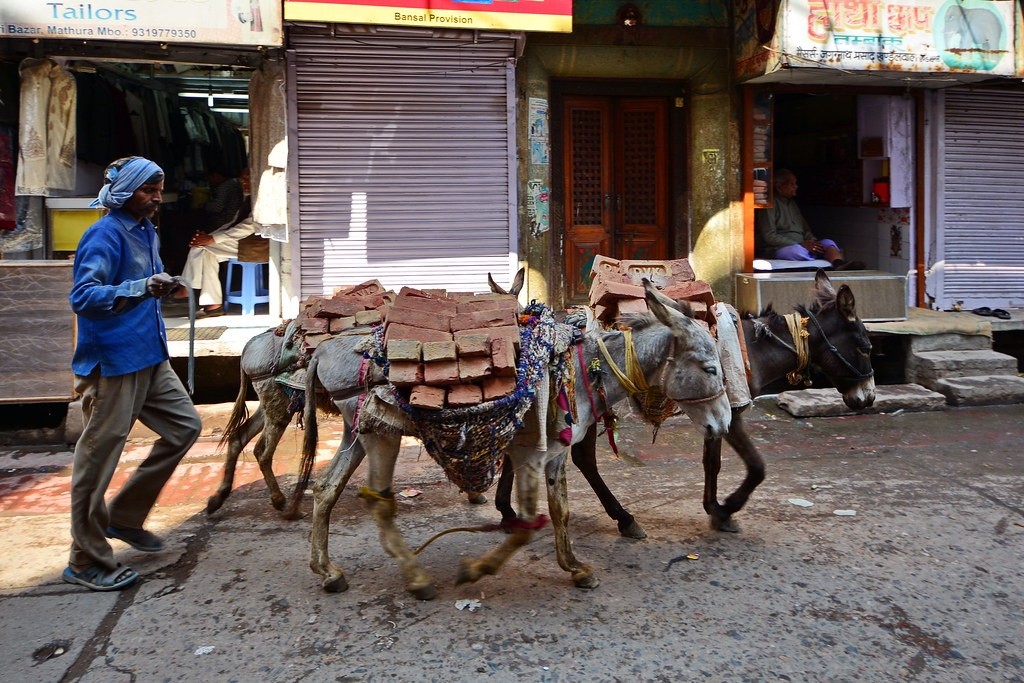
[195,267,878,601]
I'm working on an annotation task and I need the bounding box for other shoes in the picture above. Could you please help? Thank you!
[188,306,225,319]
[166,294,188,304]
[833,259,867,270]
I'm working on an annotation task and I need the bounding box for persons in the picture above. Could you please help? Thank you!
[62,157,203,590]
[757,171,864,272]
[159,167,268,322]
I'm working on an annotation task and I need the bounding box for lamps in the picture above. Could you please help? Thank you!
[207,68,214,108]
[210,107,249,114]
[178,90,249,100]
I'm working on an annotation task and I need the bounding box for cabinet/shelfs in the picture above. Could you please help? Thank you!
[782,120,862,208]
[857,93,915,209]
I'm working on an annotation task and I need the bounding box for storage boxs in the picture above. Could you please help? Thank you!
[861,137,883,157]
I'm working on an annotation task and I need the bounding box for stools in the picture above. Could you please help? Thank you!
[224,259,269,316]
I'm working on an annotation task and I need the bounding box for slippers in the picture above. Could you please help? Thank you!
[105,523,166,552]
[974,307,992,316]
[993,309,1011,319]
[62,564,138,591]
